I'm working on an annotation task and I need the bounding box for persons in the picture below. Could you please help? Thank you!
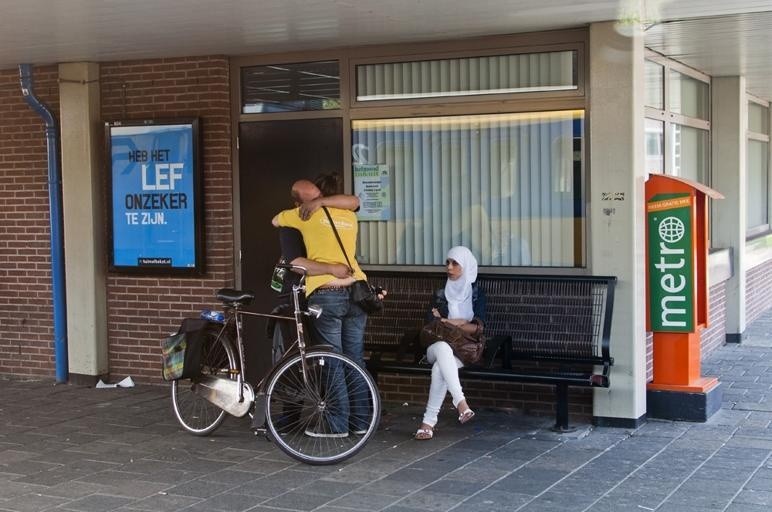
[413,245,488,441]
[269,179,361,437]
[271,169,372,439]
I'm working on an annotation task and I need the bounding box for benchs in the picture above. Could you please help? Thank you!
[296,270,619,433]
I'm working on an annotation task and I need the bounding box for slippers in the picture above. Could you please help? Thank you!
[415,426,436,440]
[457,407,476,424]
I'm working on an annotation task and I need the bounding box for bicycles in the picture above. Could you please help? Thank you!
[171,262,381,465]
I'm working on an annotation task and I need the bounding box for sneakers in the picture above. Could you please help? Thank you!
[305,423,368,438]
[278,414,300,435]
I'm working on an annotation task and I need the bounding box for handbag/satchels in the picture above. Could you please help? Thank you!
[159,317,210,381]
[208,322,238,370]
[271,253,293,294]
[350,279,386,315]
[481,334,514,372]
[418,316,485,366]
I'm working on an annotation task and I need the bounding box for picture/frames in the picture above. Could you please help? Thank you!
[102,115,205,279]
